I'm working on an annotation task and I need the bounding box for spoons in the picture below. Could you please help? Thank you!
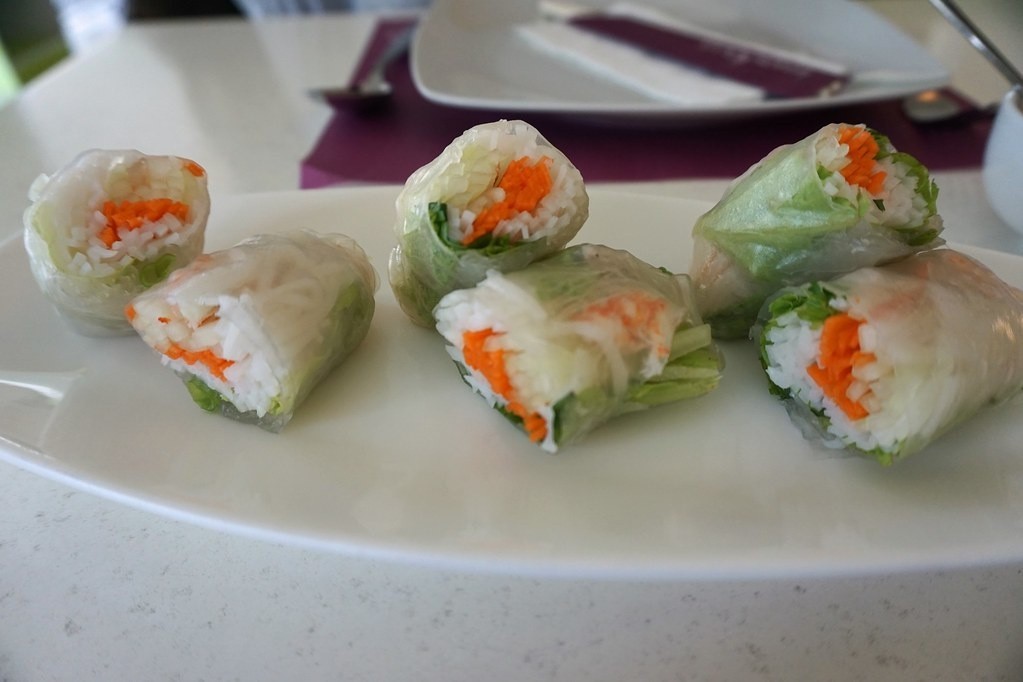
[307,22,412,118]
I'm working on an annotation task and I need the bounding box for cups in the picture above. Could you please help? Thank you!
[982,81,1023,234]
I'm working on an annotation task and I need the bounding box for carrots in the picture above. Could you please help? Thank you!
[92,124,893,443]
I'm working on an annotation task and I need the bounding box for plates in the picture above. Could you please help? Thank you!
[0,192,1023,571]
[409,0,950,132]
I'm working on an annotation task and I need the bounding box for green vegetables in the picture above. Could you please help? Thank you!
[123,128,947,465]
